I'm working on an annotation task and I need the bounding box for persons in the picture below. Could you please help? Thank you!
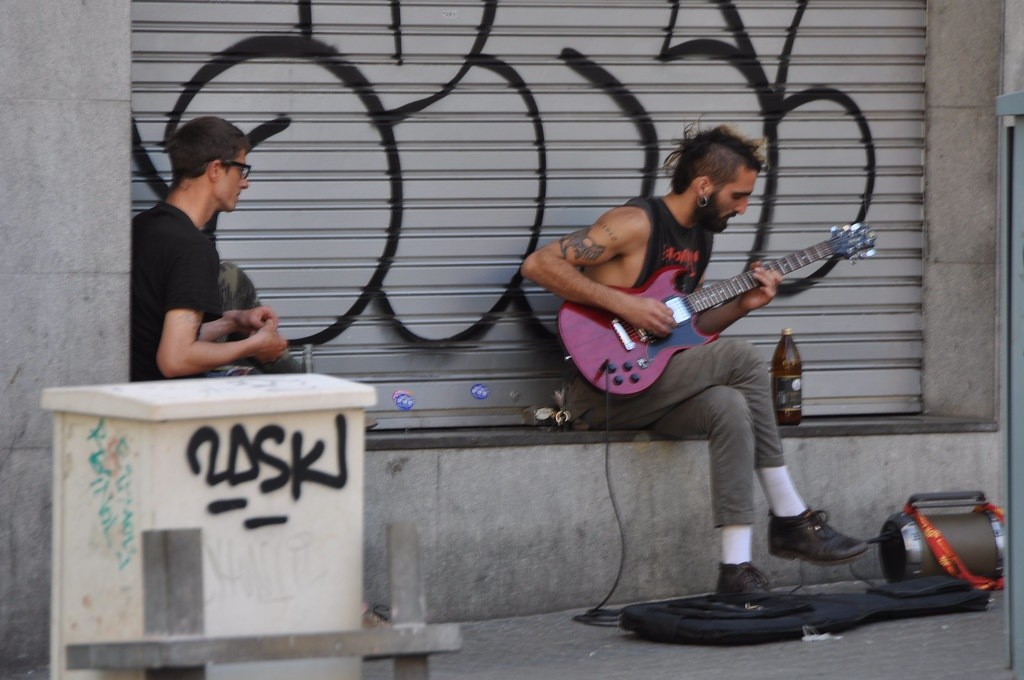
[129,116,390,661]
[521,125,871,605]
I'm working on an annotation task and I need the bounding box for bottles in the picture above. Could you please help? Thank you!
[772,329,803,427]
[302,343,315,373]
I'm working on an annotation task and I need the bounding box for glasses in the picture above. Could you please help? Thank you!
[206,159,251,180]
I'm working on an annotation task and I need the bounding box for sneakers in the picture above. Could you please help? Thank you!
[768,507,869,565]
[717,563,770,593]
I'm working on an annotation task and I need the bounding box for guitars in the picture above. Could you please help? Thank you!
[555,220,882,402]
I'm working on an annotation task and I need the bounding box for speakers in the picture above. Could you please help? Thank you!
[879,510,1005,583]
